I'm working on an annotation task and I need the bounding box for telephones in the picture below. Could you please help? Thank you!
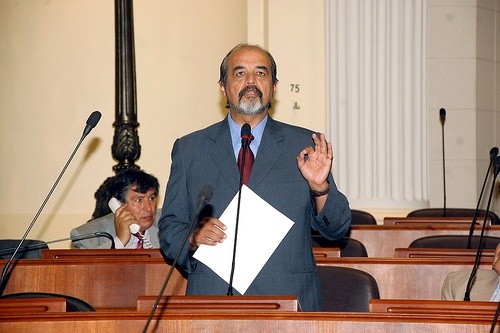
[108,197,141,234]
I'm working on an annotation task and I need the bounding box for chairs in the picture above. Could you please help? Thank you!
[351,210,377,225]
[0,292,96,311]
[0,239,49,258]
[316,266,379,313]
[341,238,369,257]
[407,234,500,249]
[407,208,500,225]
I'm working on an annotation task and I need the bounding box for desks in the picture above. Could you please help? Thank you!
[0,294,500,333]
[346,217,500,257]
[0,247,499,306]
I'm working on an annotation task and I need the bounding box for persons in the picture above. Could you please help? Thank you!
[70,169,163,250]
[441,242,500,302]
[158,42,351,312]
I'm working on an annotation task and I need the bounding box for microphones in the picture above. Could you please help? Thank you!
[227,123,252,297]
[0,111,102,296]
[439,108,448,217]
[463,147,500,302]
[142,183,216,333]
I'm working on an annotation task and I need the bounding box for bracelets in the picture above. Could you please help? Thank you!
[310,187,329,197]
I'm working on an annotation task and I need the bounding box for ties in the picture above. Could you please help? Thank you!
[137,235,144,249]
[237,133,255,186]
